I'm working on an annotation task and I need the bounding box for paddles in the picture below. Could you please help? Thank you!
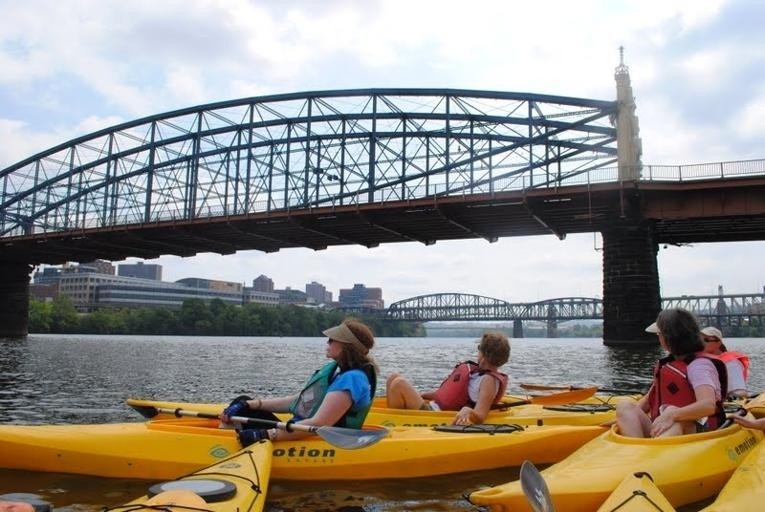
[519,460,554,512]
[157,409,390,450]
[520,382,647,398]
[490,387,598,411]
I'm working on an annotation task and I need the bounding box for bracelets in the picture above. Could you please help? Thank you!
[255,399,262,411]
[271,427,277,441]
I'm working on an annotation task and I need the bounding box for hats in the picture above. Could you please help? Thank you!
[645,322,662,335]
[700,326,723,342]
[322,322,369,354]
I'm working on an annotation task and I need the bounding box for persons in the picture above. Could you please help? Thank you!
[219,318,380,448]
[599,308,724,438]
[386,331,512,426]
[702,327,749,397]
[731,414,765,431]
